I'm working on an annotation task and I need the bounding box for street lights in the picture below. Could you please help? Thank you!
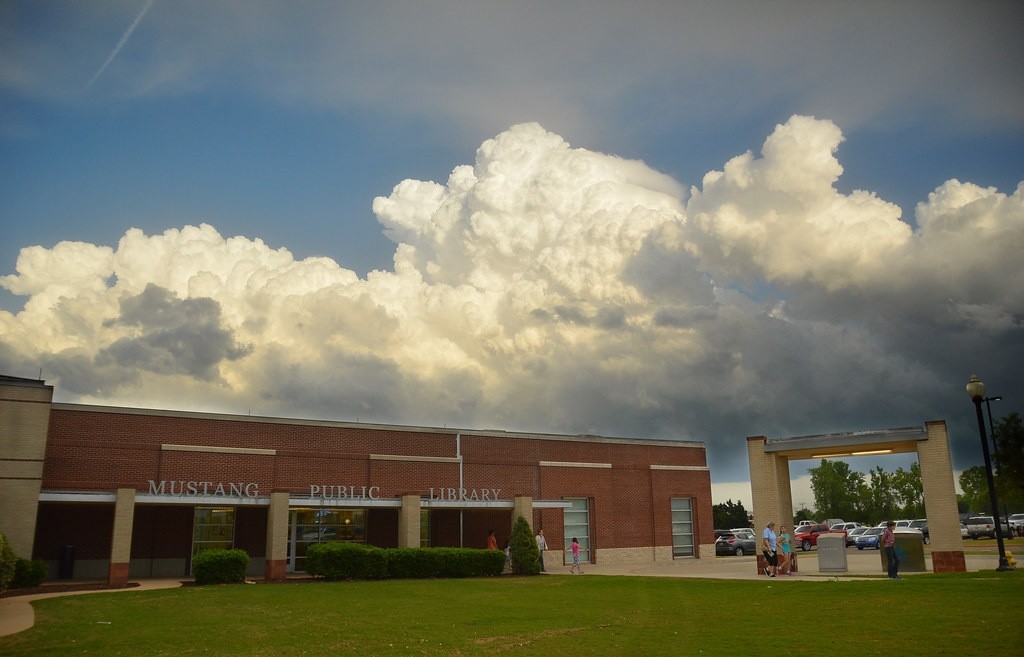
[984,395,1013,539]
[966,375,1013,571]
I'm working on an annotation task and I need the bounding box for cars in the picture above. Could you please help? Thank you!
[877,521,910,530]
[302,529,319,540]
[1004,514,1024,530]
[822,519,844,527]
[730,528,754,533]
[909,519,930,544]
[959,523,981,538]
[716,532,756,557]
[847,527,869,547]
[830,522,861,534]
[855,527,886,550]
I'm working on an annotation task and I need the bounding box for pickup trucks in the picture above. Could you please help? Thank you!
[794,521,818,529]
[794,524,847,552]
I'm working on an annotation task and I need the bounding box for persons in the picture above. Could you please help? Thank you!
[563,537,587,573]
[881,521,901,579]
[535,529,548,572]
[761,521,792,578]
[487,528,497,550]
[503,540,512,570]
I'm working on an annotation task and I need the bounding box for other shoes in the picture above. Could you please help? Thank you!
[771,575,776,578]
[764,568,771,576]
[888,576,901,580]
[787,571,791,576]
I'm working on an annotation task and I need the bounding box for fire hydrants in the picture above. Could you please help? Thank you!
[1005,550,1017,570]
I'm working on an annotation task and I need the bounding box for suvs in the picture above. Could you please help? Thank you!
[967,516,1015,540]
